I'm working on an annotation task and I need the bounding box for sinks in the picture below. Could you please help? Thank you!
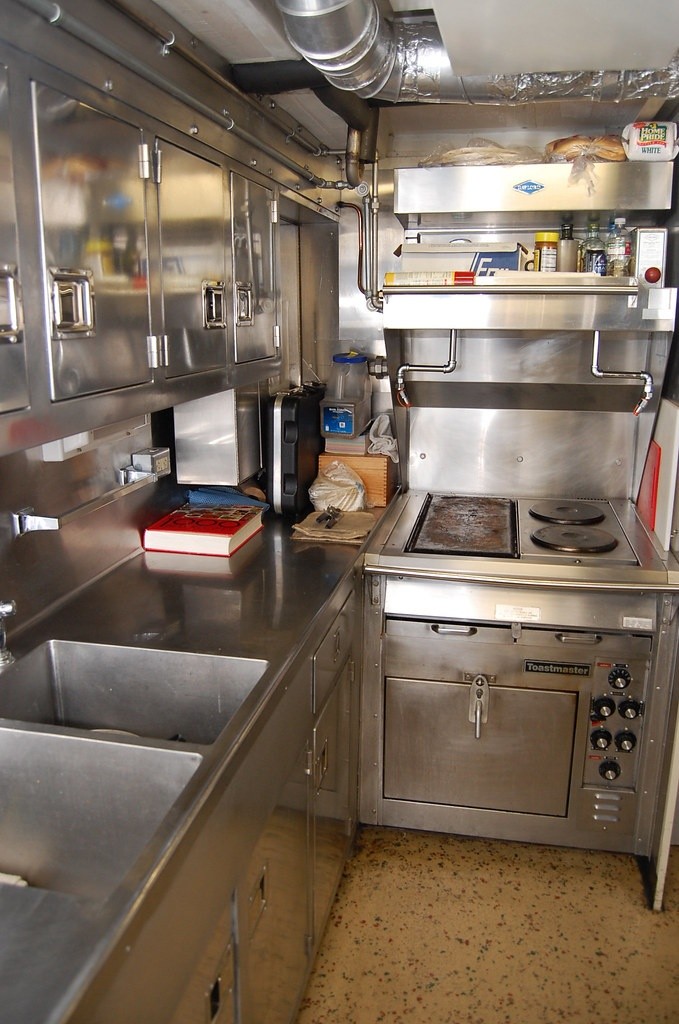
[1,636,271,744]
[1,723,206,903]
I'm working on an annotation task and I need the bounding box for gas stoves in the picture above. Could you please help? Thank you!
[383,477,678,589]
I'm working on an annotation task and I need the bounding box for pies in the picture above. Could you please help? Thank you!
[437,146,519,165]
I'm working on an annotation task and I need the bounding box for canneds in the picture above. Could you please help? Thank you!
[533,232,560,272]
[583,248,607,276]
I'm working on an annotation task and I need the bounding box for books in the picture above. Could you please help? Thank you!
[144,503,264,557]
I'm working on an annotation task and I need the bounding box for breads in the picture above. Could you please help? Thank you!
[541,134,627,162]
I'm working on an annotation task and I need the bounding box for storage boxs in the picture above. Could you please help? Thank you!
[630,225,668,290]
[391,241,527,277]
[319,396,398,507]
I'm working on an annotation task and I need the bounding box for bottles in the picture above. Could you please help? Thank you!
[607,217,632,279]
[533,232,559,272]
[578,223,607,277]
[558,224,579,273]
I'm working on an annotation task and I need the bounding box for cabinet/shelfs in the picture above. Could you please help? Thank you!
[165,641,359,1024]
[0,40,289,459]
